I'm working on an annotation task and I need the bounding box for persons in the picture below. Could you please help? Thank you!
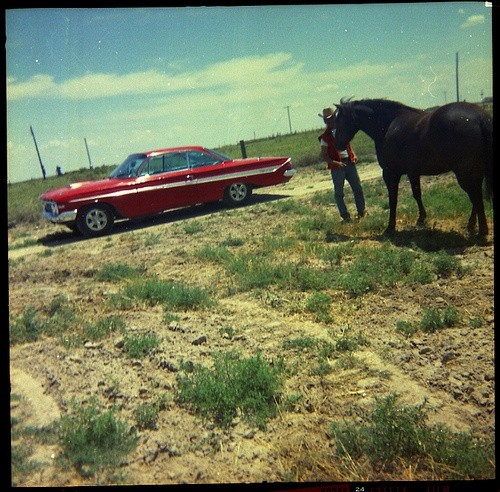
[318,106,365,225]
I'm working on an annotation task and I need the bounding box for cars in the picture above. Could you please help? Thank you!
[40,146,297,238]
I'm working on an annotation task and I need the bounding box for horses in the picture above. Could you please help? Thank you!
[333,96,493,237]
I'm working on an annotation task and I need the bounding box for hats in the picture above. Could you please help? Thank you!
[318,107,339,120]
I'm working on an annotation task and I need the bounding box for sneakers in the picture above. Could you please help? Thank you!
[344,217,354,224]
[358,212,367,223]
